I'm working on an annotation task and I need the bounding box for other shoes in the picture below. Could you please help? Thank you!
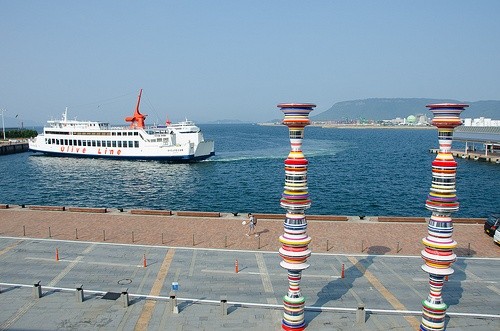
[255,234,259,236]
[245,234,249,236]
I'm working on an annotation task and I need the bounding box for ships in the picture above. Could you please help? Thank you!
[29,107,215,162]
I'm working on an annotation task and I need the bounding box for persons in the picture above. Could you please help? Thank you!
[245,212,259,237]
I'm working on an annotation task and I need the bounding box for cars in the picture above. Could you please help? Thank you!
[484,214,500,246]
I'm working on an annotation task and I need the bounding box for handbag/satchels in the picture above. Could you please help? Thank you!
[249,216,257,225]
